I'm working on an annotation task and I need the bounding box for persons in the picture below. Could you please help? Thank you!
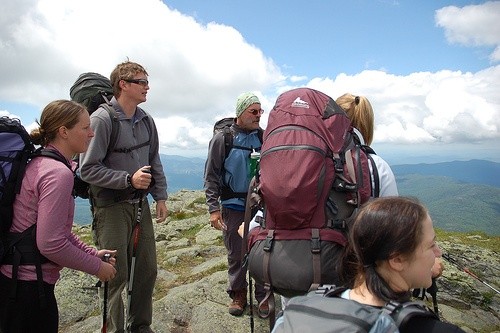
[306,198,467,333]
[204,92,275,319]
[238,93,445,316]
[0,99,118,333]
[80,56,168,333]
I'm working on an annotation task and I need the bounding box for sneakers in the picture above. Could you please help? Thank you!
[229,296,248,317]
[259,299,269,314]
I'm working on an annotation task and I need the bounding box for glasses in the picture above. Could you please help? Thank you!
[117,78,149,88]
[246,108,264,116]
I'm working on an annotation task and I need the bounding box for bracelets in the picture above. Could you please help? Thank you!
[127,173,133,188]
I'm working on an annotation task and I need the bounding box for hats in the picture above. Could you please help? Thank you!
[235,91,261,117]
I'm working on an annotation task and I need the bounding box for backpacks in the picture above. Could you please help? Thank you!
[241,89,380,297]
[70,72,150,199]
[0,117,76,267]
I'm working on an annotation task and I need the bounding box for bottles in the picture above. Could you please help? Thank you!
[250,149,260,180]
